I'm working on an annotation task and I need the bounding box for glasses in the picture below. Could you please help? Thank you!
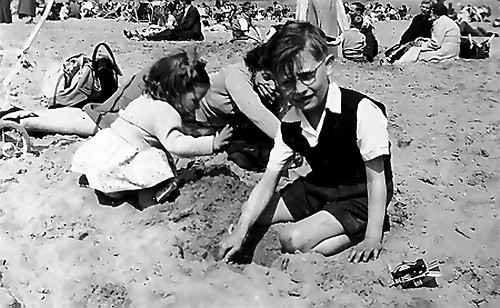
[278,58,324,91]
[258,67,275,83]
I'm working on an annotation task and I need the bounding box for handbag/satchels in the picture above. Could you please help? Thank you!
[48,43,123,110]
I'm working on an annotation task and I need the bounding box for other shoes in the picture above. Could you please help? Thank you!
[104,192,124,199]
[138,190,157,208]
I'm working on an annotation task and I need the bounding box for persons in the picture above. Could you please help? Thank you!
[0,0,295,42]
[70,48,233,210]
[219,20,394,263]
[0,43,291,140]
[296,0,500,65]
